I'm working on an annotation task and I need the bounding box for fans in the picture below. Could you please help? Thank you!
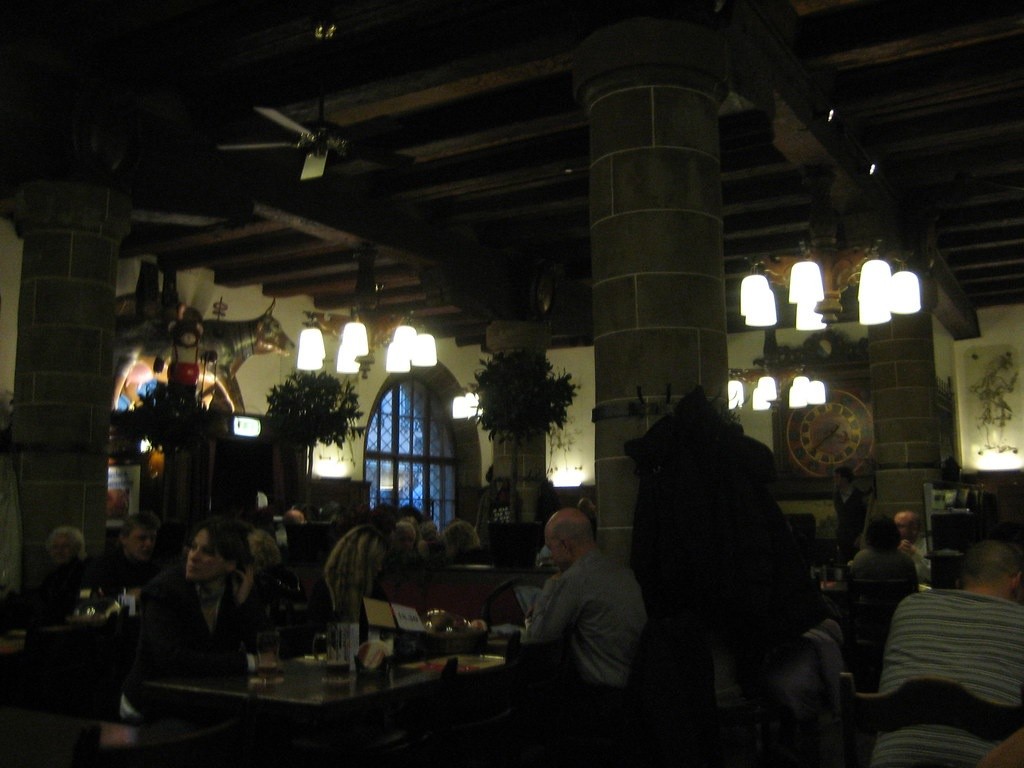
[215,9,417,181]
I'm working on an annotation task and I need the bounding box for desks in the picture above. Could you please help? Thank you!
[142,647,505,768]
[0,704,184,768]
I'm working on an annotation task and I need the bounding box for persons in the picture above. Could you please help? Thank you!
[517,498,646,702]
[22,500,482,712]
[831,464,866,553]
[121,515,279,718]
[846,510,1024,767]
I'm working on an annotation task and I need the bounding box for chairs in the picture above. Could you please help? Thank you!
[844,567,921,645]
[712,653,815,768]
[15,612,121,721]
[839,670,1024,768]
[559,602,727,768]
[71,694,263,768]
[435,631,524,768]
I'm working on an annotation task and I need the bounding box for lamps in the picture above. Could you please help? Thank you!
[727,329,828,412]
[296,281,438,380]
[738,217,922,333]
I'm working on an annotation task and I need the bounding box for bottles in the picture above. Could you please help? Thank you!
[826,568,834,581]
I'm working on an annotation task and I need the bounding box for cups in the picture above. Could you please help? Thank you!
[256,631,281,695]
[312,622,359,672]
[932,489,971,509]
[119,594,137,616]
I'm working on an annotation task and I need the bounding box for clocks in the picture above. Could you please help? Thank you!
[786,387,875,478]
